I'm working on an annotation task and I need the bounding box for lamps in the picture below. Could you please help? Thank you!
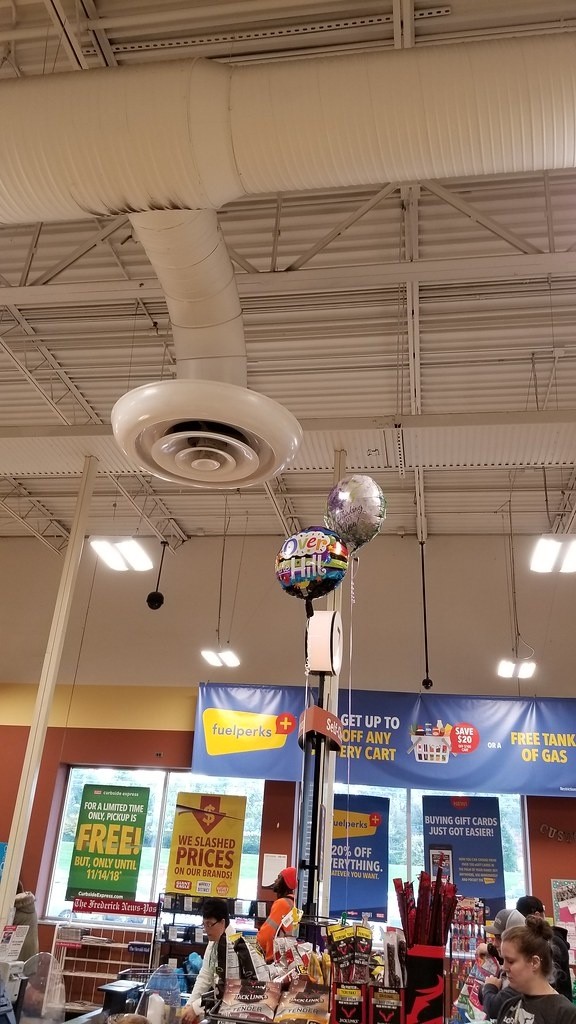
[87,533,153,573]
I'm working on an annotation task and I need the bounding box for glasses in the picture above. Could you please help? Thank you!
[200,917,225,929]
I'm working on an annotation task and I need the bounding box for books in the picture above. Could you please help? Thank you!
[58,925,115,945]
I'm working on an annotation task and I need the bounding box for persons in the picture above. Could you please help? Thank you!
[481,909,526,1019]
[179,899,236,1024]
[475,895,573,1003]
[256,867,297,965]
[496,915,576,1024]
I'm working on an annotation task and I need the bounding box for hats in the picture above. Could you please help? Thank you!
[516,896,545,917]
[281,867,297,890]
[482,909,526,936]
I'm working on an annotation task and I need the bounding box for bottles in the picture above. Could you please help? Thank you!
[146,990,164,1024]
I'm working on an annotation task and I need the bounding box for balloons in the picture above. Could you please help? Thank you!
[274,526,348,619]
[324,475,387,562]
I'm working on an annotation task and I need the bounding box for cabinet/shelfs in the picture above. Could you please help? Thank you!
[40,922,153,1018]
